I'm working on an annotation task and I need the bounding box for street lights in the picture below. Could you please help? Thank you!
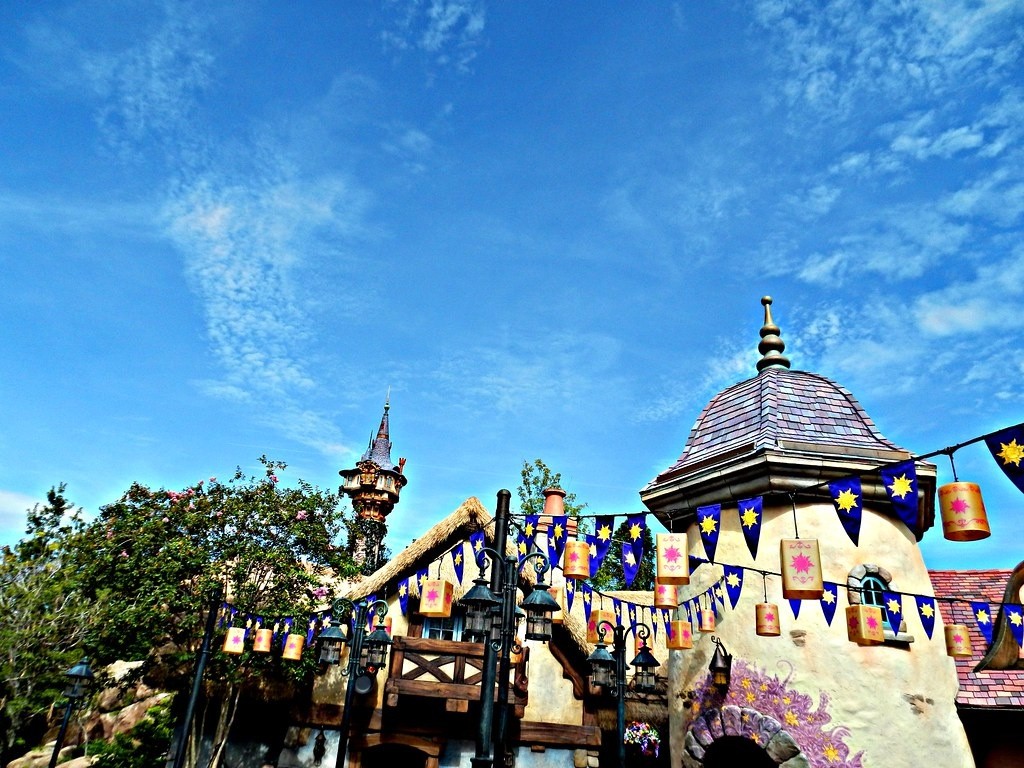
[458,546,562,768]
[316,597,395,768]
[585,620,662,768]
[48,655,97,768]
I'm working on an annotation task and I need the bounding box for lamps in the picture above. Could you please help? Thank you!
[634,576,714,661]
[755,571,781,636]
[937,451,991,542]
[656,511,690,585]
[419,556,455,619]
[846,591,885,645]
[221,625,305,660]
[586,595,617,645]
[779,492,824,599]
[945,600,973,658]
[563,516,590,579]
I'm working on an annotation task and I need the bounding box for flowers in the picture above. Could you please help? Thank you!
[622,721,661,757]
